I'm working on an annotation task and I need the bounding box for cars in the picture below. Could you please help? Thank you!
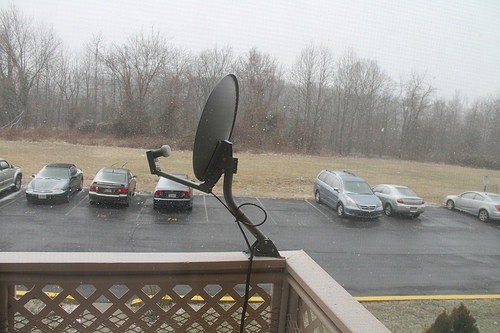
[88,168,137,207]
[153,174,193,212]
[441,190,500,222]
[26,163,84,205]
[371,184,426,218]
[0,160,22,197]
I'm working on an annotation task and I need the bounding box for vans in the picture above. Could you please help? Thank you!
[313,169,384,220]
